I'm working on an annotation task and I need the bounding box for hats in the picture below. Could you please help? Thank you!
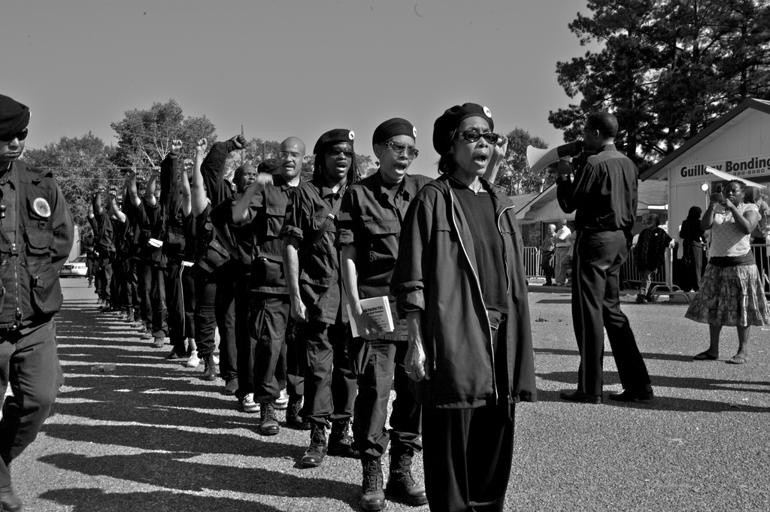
[432,102,494,156]
[313,129,355,154]
[372,118,417,145]
[256,158,279,173]
[0,95,30,137]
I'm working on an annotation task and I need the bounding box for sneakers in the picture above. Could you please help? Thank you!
[635,294,647,304]
[543,282,565,286]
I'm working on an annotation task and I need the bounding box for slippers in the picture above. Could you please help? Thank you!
[728,355,753,364]
[693,350,719,360]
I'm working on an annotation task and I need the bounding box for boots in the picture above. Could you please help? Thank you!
[95,297,199,368]
[200,355,311,435]
[360,456,385,511]
[386,449,427,506]
[300,426,327,467]
[327,425,360,459]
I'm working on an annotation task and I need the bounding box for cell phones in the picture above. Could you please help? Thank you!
[716,183,723,203]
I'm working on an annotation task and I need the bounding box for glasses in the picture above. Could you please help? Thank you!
[456,130,498,144]
[384,141,420,161]
[327,147,355,159]
[0,129,28,141]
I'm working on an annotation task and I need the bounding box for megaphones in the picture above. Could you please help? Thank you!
[527,140,583,176]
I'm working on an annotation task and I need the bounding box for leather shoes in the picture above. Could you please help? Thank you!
[608,385,654,402]
[560,390,603,404]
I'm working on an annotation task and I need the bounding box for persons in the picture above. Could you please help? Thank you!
[0,94,75,512]
